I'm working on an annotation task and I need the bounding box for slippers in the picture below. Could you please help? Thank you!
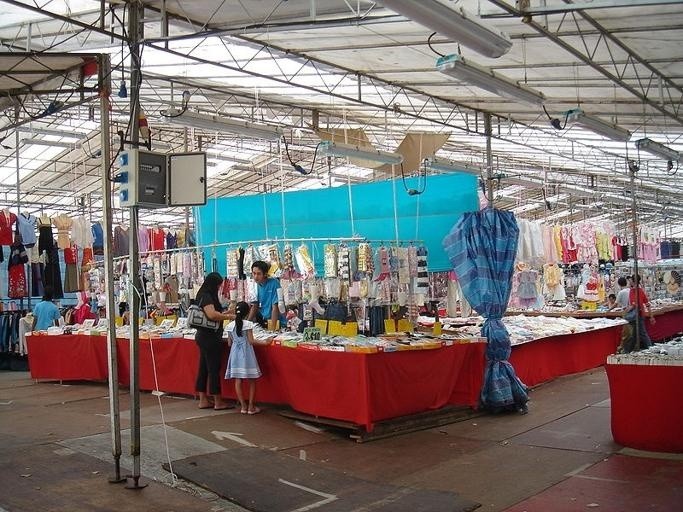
[199,401,235,410]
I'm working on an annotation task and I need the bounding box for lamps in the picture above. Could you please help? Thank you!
[632,106,678,161]
[419,113,483,179]
[492,150,632,206]
[374,0,516,60]
[163,57,285,141]
[432,35,548,109]
[320,95,405,166]
[566,58,632,144]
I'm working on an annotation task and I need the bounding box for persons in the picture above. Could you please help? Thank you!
[603,274,656,353]
[246,260,283,333]
[224,302,273,415]
[1,207,105,254]
[114,223,187,255]
[192,271,236,410]
[30,294,60,331]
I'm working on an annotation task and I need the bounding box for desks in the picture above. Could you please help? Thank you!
[24,303,683,453]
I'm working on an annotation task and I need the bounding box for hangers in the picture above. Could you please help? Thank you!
[87,237,425,270]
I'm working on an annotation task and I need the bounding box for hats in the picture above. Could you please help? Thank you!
[627,275,641,284]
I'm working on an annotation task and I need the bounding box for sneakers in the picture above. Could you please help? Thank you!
[240,405,261,415]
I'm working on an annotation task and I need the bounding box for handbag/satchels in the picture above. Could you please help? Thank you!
[624,304,640,321]
[186,304,220,330]
